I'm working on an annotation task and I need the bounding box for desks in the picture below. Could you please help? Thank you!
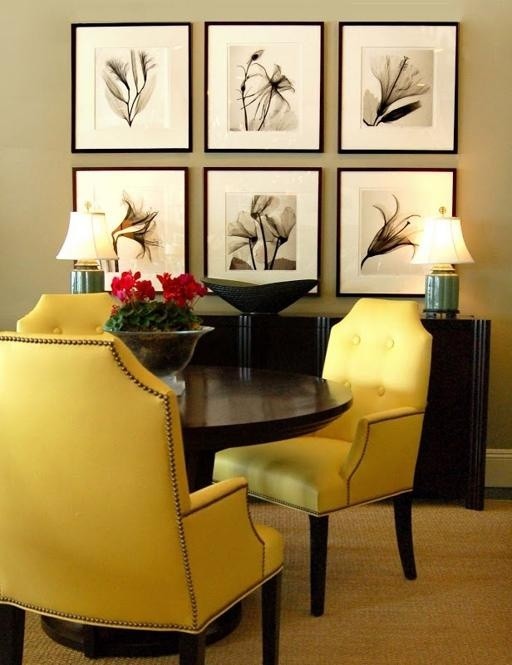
[40,369,353,655]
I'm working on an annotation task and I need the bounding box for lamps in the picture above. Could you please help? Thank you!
[411,217,474,317]
[56,211,118,294]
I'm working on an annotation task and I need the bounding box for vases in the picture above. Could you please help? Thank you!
[103,322,214,397]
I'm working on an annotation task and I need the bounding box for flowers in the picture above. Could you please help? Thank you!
[107,271,207,330]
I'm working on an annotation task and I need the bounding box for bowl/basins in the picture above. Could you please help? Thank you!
[196,276,320,315]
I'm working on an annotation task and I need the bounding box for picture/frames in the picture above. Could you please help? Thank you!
[72,168,188,294]
[204,21,324,152]
[338,21,460,152]
[72,23,193,152]
[203,166,320,298]
[337,167,456,298]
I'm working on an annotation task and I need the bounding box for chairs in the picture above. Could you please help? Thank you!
[0,332,285,665]
[214,298,432,617]
[16,292,112,333]
